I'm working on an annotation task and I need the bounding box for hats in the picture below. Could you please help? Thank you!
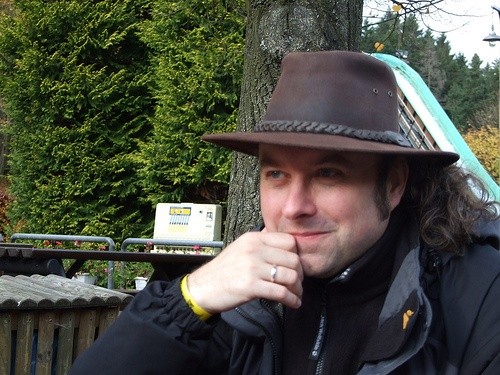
[201,50,460,179]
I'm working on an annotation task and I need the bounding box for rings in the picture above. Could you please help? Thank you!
[270,264,277,282]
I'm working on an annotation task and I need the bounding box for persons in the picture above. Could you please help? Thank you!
[59,51,500,375]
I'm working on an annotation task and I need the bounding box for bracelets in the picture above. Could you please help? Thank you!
[179,273,215,324]
